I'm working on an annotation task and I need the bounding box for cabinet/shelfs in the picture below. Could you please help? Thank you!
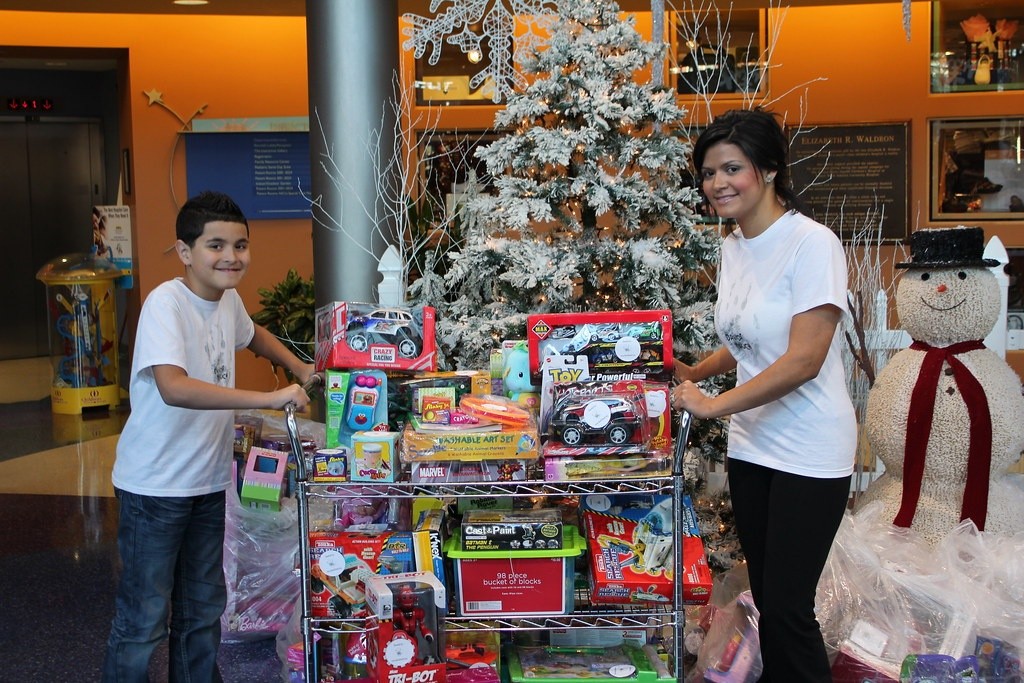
[283,369,700,680]
[664,0,770,100]
[916,0,1024,248]
[410,12,516,107]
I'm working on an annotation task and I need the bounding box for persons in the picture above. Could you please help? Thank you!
[102,192,317,683]
[670,107,859,682]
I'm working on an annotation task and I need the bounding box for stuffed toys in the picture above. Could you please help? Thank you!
[501,349,541,419]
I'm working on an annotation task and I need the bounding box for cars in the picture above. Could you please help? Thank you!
[219,297,718,683]
